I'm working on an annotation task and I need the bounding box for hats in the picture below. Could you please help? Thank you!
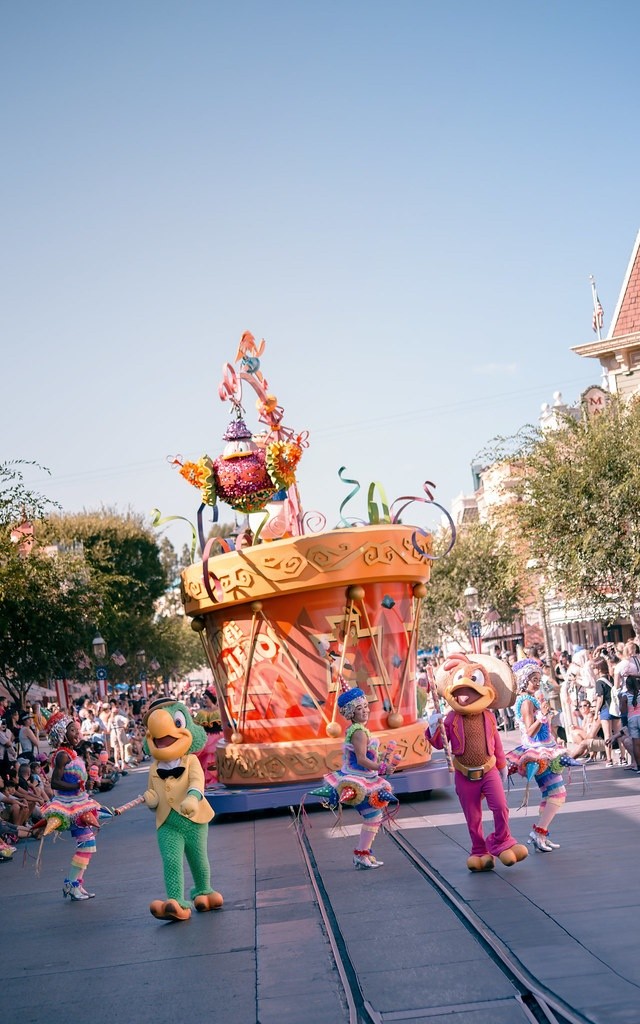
[203,685,218,705]
[22,715,35,722]
[513,660,543,693]
[338,688,368,720]
[45,713,75,747]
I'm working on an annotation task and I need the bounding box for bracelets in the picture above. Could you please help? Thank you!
[620,730,625,736]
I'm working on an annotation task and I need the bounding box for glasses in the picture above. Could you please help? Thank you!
[578,704,589,708]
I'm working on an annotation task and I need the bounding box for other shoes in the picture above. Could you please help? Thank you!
[122,764,130,770]
[624,764,637,771]
[586,758,596,763]
[605,759,613,767]
[613,759,627,766]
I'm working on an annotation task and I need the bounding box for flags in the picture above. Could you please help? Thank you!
[111,651,126,666]
[137,651,146,663]
[150,658,160,673]
[76,651,89,669]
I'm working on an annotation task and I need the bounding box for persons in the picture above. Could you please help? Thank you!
[489,642,619,743]
[307,687,399,869]
[28,717,114,900]
[605,725,638,771]
[193,687,223,735]
[614,642,640,693]
[622,676,640,774]
[592,657,627,767]
[0,679,214,863]
[506,658,588,852]
[570,700,605,762]
[615,643,628,660]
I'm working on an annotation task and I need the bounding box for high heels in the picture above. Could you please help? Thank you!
[527,831,560,852]
[353,854,384,870]
[62,883,96,901]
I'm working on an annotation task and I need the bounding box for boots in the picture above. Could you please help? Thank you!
[505,715,515,731]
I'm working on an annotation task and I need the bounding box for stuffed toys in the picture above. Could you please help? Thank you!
[425,653,528,872]
[138,697,224,920]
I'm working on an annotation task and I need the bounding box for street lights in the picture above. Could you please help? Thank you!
[526,557,561,711]
[136,649,149,699]
[92,632,107,701]
[464,580,483,654]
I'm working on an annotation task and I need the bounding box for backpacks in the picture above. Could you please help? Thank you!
[596,675,628,717]
